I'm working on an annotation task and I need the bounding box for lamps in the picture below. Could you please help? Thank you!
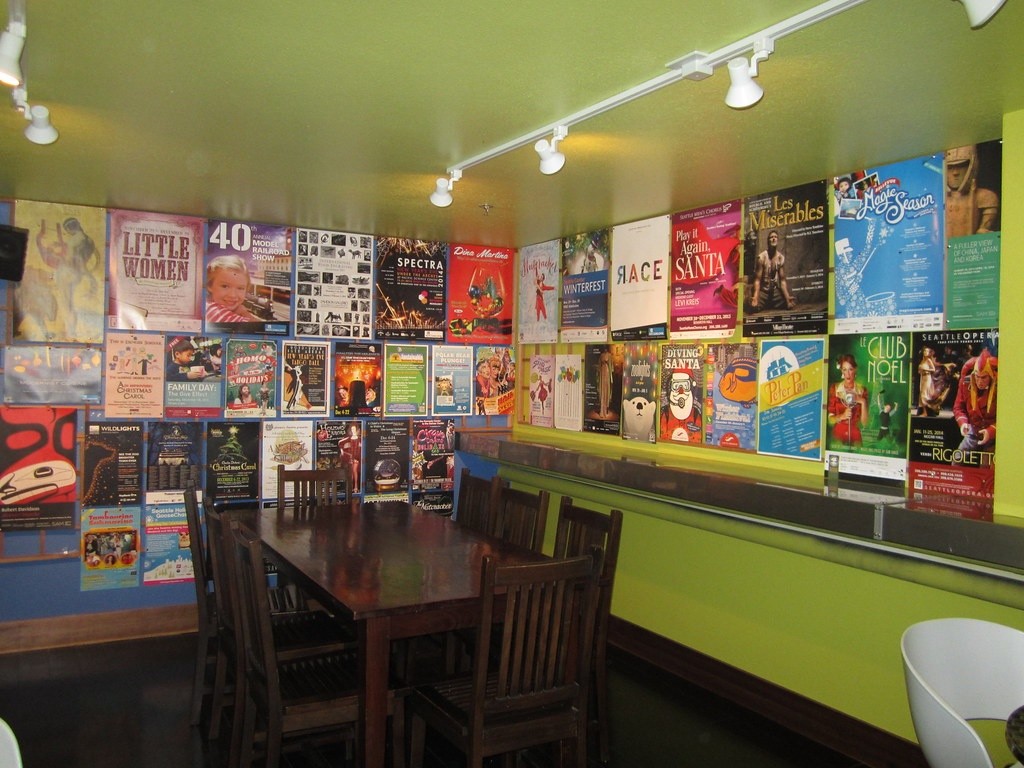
[959,0,1008,29]
[724,37,774,108]
[0,0,61,146]
[535,125,569,175]
[428,168,462,208]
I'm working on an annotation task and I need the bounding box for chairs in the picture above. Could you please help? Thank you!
[409,467,623,768]
[183,465,405,768]
[900,618,1024,768]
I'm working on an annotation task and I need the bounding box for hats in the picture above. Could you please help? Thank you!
[968,349,995,412]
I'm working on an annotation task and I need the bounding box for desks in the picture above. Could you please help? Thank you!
[219,500,577,768]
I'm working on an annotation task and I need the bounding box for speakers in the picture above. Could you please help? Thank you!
[0,223,29,281]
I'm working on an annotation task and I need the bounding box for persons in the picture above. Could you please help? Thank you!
[876,389,898,441]
[750,230,797,312]
[828,353,869,447]
[944,145,998,235]
[233,380,270,415]
[834,176,873,206]
[365,388,376,406]
[475,349,516,398]
[916,347,942,418]
[336,385,349,407]
[950,346,998,468]
[165,340,222,382]
[534,263,558,323]
[205,254,262,322]
